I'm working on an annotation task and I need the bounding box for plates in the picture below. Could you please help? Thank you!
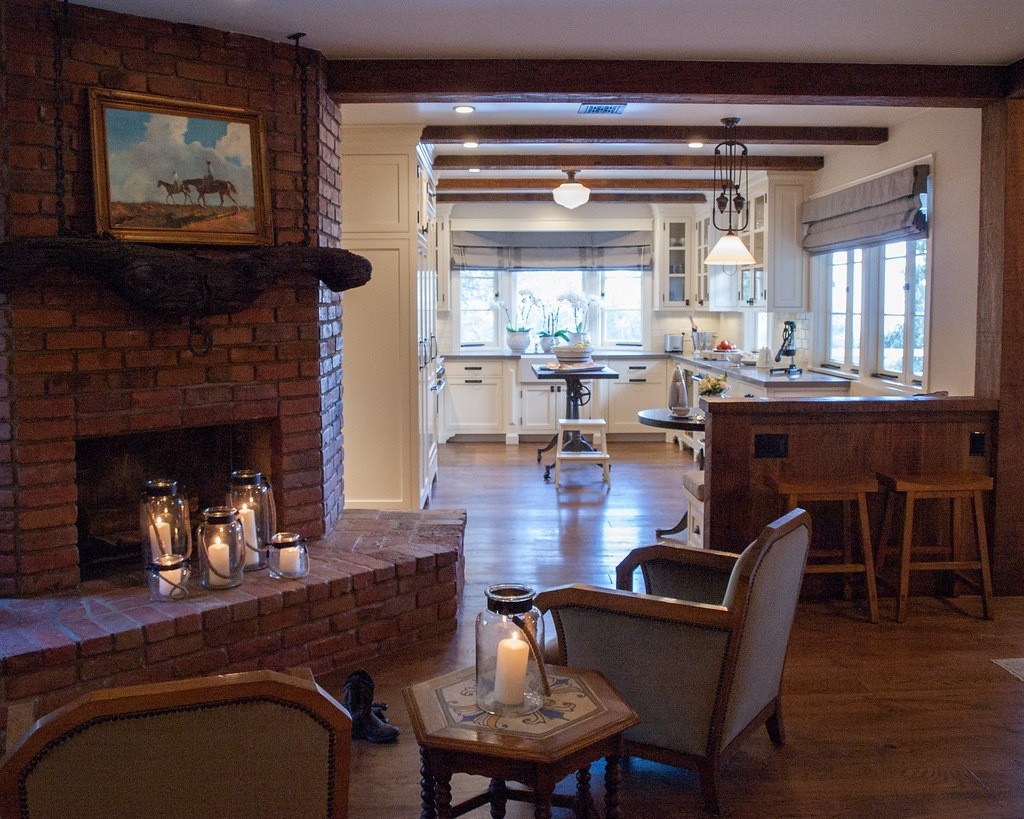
[741,360,757,365]
[670,414,693,417]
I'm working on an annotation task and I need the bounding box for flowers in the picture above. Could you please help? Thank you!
[696,371,734,395]
[488,282,607,342]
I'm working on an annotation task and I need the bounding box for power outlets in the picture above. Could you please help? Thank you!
[970,431,985,457]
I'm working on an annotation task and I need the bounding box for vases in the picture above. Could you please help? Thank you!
[507,330,532,353]
[539,336,560,352]
[565,331,593,346]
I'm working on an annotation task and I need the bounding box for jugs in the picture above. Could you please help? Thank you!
[756,346,774,369]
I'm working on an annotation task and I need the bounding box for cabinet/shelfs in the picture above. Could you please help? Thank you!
[333,127,853,512]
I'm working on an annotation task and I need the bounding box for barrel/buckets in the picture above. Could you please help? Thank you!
[692,331,711,351]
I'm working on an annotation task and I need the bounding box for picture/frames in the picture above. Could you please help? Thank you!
[83,80,275,251]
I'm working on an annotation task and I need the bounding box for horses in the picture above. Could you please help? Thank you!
[156,179,241,209]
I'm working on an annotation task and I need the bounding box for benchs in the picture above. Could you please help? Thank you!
[680,466,705,549]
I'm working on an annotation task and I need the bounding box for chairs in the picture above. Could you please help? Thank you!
[531,501,816,819]
[0,645,358,819]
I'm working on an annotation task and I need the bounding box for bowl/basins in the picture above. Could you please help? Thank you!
[553,346,592,365]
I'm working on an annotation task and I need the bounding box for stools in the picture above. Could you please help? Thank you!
[550,415,613,491]
[766,469,886,623]
[870,467,1002,624]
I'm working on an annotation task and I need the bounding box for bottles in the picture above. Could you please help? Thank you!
[266,531,311,579]
[148,555,190,603]
[476,582,547,718]
[226,469,276,573]
[198,506,246,589]
[668,367,689,410]
[139,478,193,565]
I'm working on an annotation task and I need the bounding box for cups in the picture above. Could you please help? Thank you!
[672,406,690,417]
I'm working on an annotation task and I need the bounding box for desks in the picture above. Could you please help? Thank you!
[634,405,705,540]
[530,362,620,484]
[399,650,640,819]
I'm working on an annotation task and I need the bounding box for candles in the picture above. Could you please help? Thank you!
[279,545,302,572]
[207,536,234,584]
[155,560,184,594]
[149,520,174,575]
[489,631,534,707]
[238,503,261,568]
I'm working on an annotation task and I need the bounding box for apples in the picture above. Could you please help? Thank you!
[716,340,736,350]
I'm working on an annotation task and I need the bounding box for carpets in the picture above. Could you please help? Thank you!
[989,657,1024,684]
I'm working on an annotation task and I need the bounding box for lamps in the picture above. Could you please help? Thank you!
[550,166,597,211]
[701,114,760,268]
